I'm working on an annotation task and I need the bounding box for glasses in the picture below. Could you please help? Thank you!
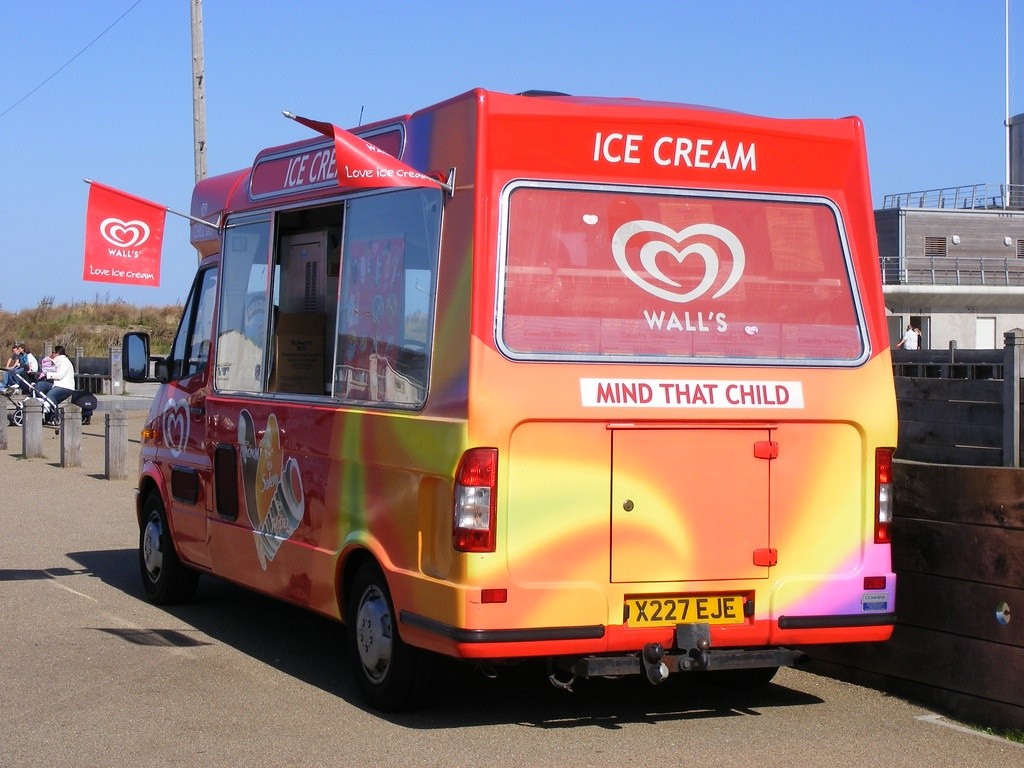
[52,351,56,354]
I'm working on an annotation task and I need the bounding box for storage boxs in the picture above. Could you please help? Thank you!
[266,312,325,394]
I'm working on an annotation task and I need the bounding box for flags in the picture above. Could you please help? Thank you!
[84,181,168,287]
[295,116,442,188]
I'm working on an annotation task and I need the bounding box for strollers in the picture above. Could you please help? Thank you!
[0,373,63,428]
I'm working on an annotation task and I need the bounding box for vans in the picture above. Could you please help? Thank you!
[118,85,904,718]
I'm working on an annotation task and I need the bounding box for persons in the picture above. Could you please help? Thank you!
[0,344,39,394]
[35,346,75,408]
[895,325,921,350]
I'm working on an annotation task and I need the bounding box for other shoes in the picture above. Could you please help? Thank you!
[10,383,20,389]
